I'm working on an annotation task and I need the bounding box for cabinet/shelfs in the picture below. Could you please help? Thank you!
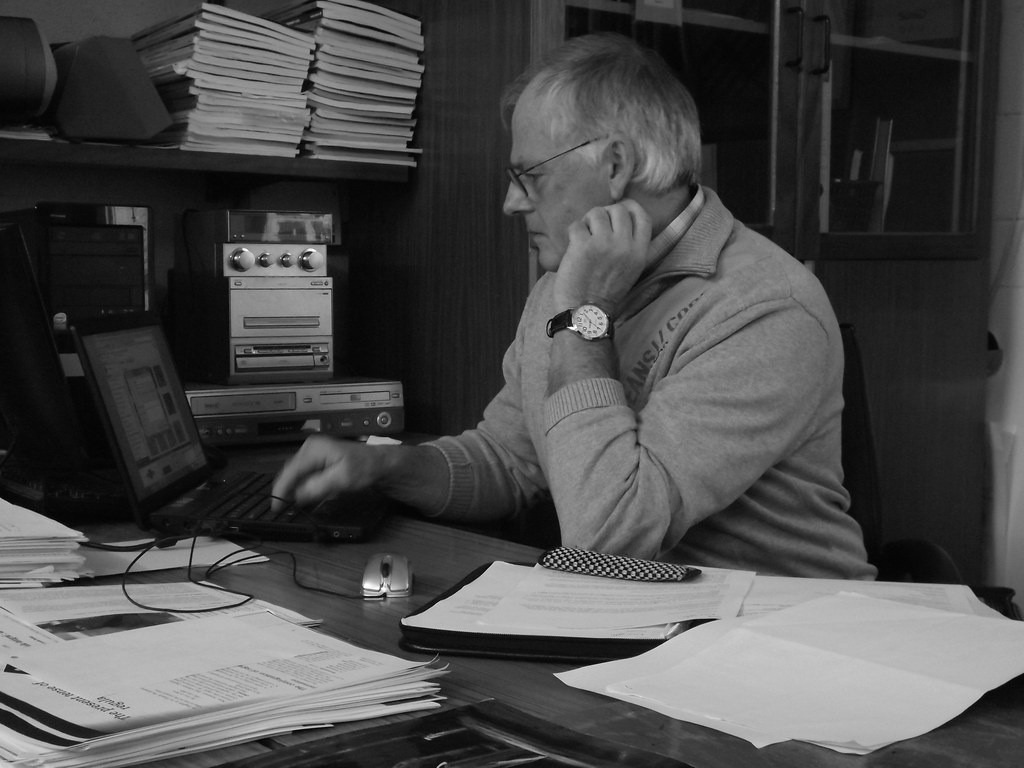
[348,0,1004,571]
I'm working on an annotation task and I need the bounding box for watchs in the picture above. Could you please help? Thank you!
[543,303,617,343]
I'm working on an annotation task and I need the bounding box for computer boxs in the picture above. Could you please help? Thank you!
[25,224,145,322]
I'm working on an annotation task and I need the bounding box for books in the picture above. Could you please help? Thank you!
[131,0,426,167]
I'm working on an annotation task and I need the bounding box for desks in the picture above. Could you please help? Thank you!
[64,512,1024,768]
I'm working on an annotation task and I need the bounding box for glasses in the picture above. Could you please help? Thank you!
[505,136,606,198]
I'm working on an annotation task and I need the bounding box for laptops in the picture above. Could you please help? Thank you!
[70,312,393,541]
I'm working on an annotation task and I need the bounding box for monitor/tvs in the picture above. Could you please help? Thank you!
[0,223,72,443]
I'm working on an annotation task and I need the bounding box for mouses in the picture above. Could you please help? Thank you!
[363,550,414,597]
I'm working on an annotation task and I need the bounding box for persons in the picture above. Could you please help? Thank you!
[269,33,881,582]
